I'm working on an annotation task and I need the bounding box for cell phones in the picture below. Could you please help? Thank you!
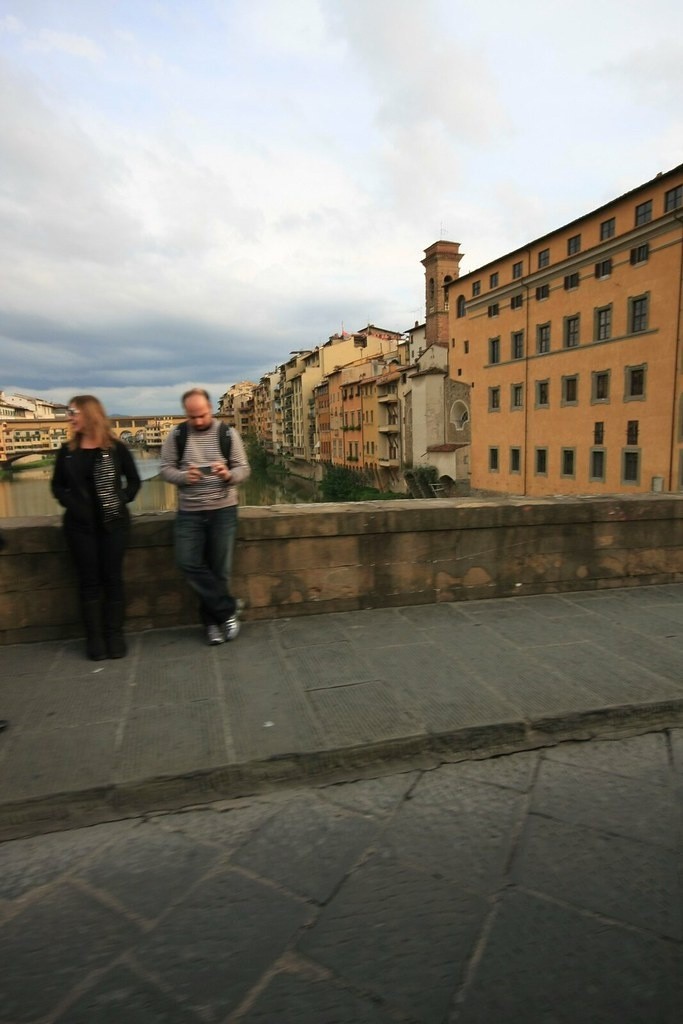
[197,466,211,476]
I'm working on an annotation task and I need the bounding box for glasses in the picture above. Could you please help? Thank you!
[65,408,80,417]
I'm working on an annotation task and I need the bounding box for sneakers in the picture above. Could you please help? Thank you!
[207,598,246,645]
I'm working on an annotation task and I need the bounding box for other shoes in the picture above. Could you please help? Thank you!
[88,636,126,661]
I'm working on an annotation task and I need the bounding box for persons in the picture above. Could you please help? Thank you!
[49,394,144,661]
[158,387,253,645]
